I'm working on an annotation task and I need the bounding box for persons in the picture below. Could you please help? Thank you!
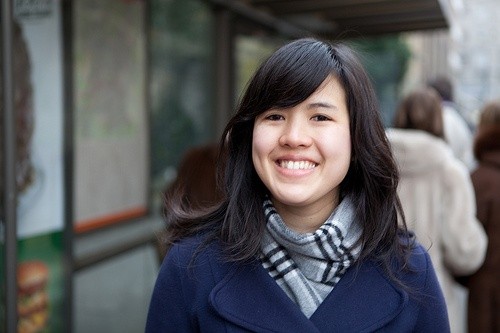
[146,38,450,333]
[384,75,500,333]
